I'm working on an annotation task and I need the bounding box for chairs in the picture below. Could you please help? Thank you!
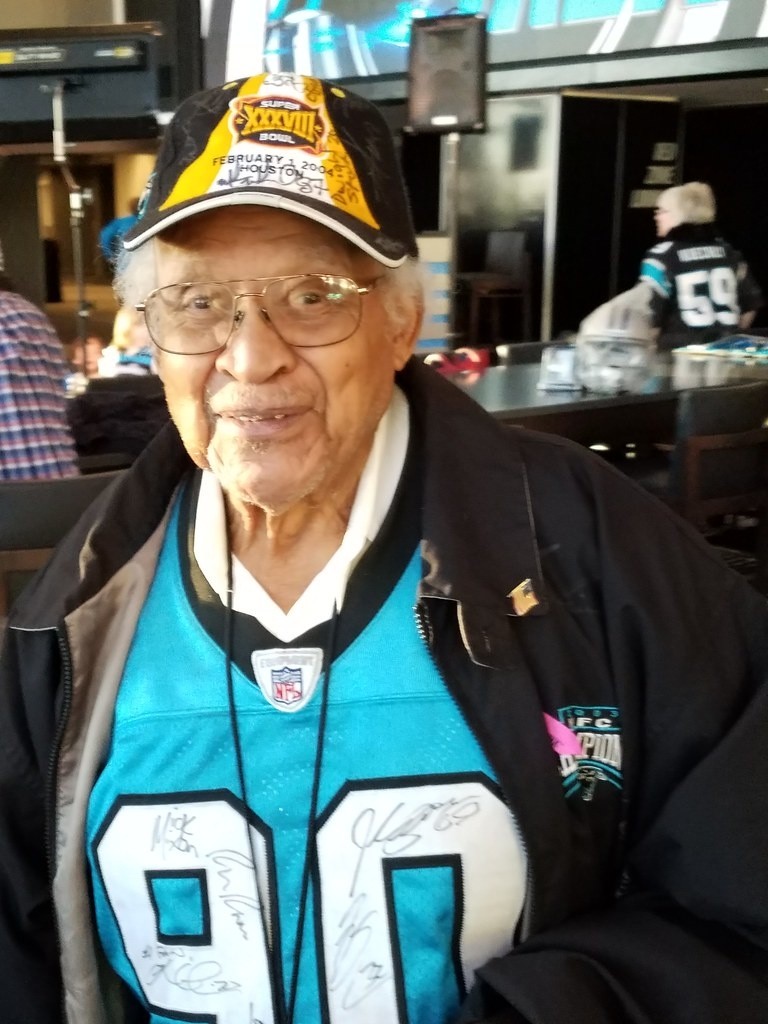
[457,228,535,351]
[1,471,126,622]
[619,379,768,551]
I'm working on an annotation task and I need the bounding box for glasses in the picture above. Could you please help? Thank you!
[132,274,391,357]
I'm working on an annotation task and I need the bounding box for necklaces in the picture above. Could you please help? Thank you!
[222,494,337,1024]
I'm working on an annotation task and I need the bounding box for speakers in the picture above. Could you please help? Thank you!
[404,12,488,137]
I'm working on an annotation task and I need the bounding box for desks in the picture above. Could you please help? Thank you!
[437,339,767,469]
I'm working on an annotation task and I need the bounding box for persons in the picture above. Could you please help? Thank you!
[0,78,768,1024]
[0,290,82,481]
[638,183,756,350]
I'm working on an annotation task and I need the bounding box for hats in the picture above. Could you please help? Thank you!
[122,70,418,272]
